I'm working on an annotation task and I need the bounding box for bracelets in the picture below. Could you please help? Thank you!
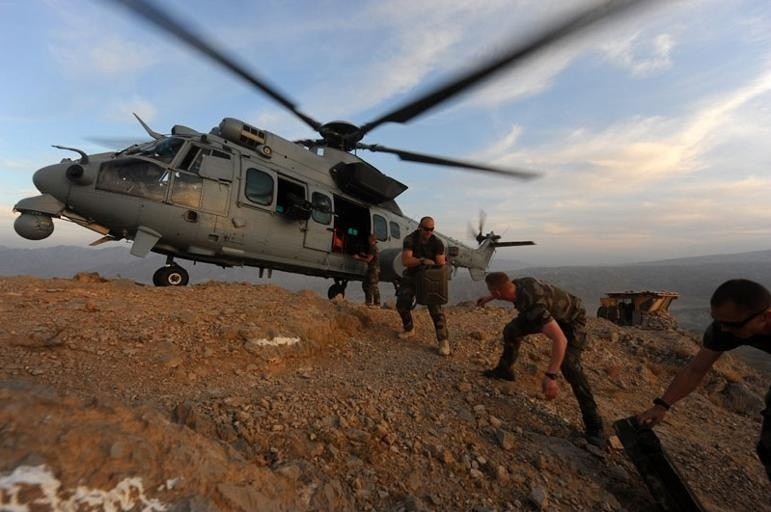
[420,257,426,264]
[545,372,558,380]
[654,397,670,410]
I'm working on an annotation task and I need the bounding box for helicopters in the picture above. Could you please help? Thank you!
[11,0,649,289]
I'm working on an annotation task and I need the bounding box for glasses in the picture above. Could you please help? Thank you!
[419,224,434,232]
[712,307,768,330]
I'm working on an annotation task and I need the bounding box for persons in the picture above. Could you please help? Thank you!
[396,216,451,357]
[476,271,613,449]
[635,277,771,480]
[353,235,382,310]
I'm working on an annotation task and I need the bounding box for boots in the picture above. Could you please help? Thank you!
[482,365,516,381]
[398,328,415,339]
[438,339,450,355]
[582,415,607,446]
